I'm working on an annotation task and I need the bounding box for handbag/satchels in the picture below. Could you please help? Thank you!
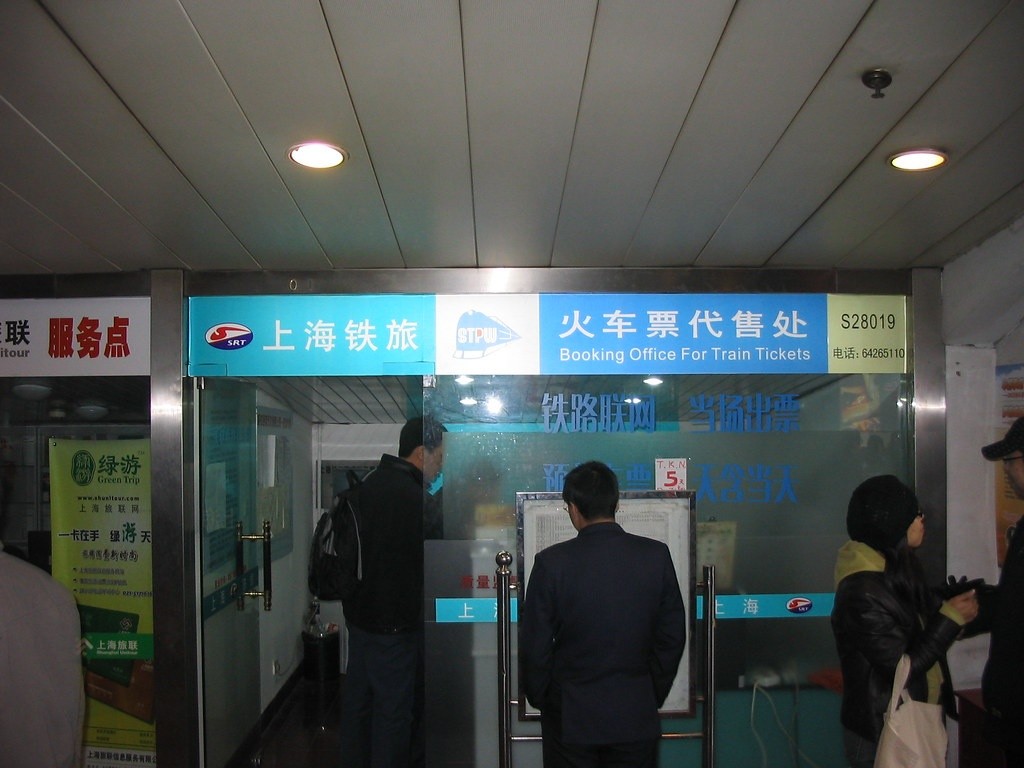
[874,655,950,768]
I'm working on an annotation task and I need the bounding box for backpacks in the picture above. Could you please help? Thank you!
[307,471,364,601]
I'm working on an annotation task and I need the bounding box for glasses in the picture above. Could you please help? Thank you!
[1002,454,1024,465]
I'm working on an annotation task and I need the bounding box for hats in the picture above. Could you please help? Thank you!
[845,474,919,546]
[982,418,1024,460]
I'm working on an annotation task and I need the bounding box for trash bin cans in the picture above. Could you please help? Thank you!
[300,623,342,682]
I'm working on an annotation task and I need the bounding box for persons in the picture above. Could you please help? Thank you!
[832,476,978,768]
[521,462,687,768]
[341,417,448,768]
[954,416,1023,768]
[0,542,84,768]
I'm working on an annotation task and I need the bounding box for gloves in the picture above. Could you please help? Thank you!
[939,575,977,603]
